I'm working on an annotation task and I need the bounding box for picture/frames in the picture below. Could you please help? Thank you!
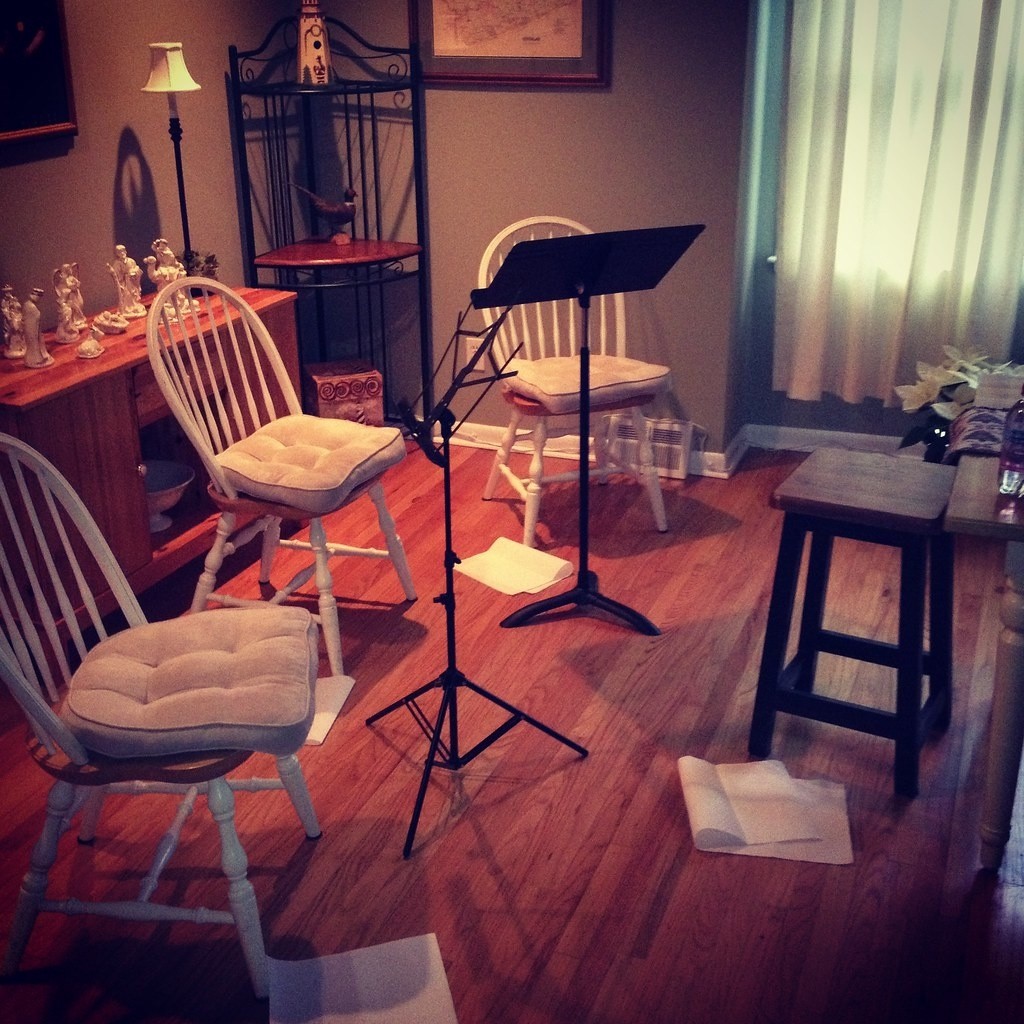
[0,0,78,149]
[407,0,611,89]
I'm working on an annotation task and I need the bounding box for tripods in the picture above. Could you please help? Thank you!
[365,299,588,862]
[471,221,705,637]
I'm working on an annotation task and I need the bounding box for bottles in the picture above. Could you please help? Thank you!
[997,383,1024,497]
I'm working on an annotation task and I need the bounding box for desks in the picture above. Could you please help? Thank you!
[945,361,1024,870]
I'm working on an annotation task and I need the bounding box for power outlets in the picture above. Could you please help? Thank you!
[465,338,486,371]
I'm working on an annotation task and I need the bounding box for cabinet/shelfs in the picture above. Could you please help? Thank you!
[0,286,303,687]
[229,0,444,469]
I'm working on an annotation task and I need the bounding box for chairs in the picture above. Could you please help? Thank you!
[0,431,322,1003]
[478,215,675,548]
[146,275,419,678]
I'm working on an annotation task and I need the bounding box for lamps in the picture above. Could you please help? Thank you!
[140,42,222,297]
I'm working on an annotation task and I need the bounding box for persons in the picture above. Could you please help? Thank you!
[22,288,54,365]
[151,239,175,266]
[51,264,91,340]
[1,284,26,352]
[107,245,145,313]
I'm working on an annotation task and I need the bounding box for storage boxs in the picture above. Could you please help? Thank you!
[304,358,384,428]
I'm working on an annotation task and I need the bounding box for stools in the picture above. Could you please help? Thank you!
[748,444,960,799]
